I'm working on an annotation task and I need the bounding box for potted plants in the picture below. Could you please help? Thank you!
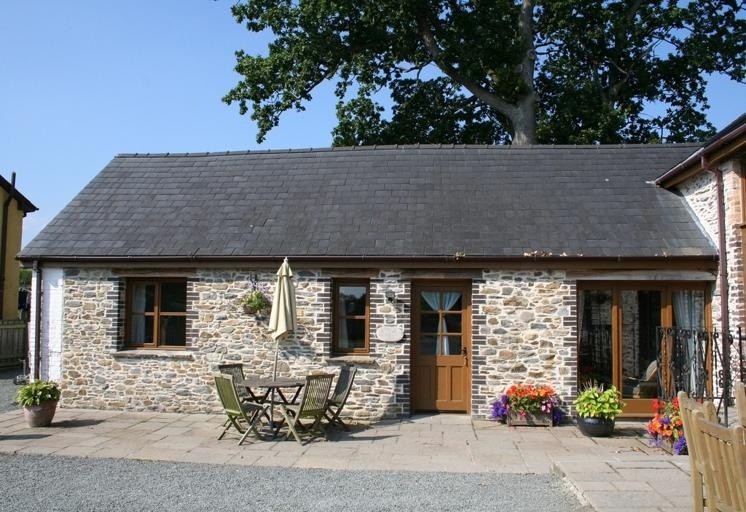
[12,379,61,427]
[235,288,268,321]
[572,379,626,436]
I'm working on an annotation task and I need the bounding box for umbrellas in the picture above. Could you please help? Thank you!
[266,256,298,431]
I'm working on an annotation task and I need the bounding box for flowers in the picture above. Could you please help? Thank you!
[492,383,564,426]
[647,396,704,455]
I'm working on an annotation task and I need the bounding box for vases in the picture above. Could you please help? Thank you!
[657,433,673,455]
[504,407,553,427]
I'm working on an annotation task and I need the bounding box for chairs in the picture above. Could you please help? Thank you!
[677,382,746,511]
[213,361,357,447]
